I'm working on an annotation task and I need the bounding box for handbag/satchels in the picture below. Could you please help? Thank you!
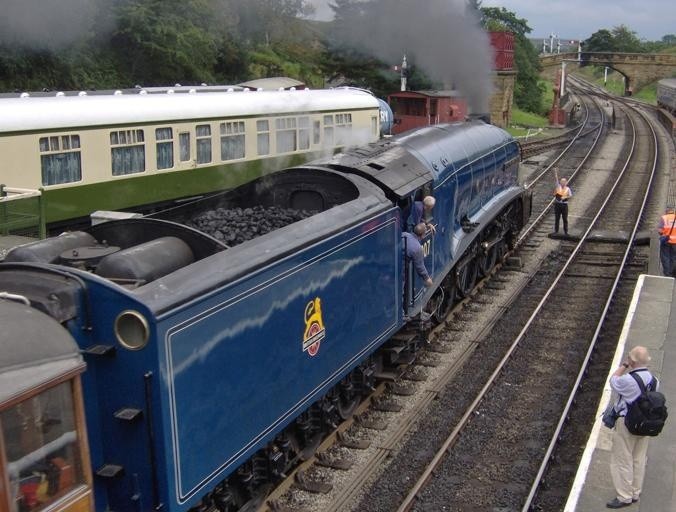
[659,236,669,243]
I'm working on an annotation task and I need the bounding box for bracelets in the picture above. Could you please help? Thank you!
[622,362,629,369]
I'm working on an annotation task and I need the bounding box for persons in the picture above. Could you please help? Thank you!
[401,223,432,315]
[414,195,437,235]
[604,345,660,509]
[552,177,572,234]
[657,203,676,278]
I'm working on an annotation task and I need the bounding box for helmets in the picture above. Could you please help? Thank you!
[414,224,426,234]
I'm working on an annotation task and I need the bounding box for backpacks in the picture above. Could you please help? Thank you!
[625,372,667,437]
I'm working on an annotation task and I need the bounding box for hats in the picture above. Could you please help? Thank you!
[667,204,674,208]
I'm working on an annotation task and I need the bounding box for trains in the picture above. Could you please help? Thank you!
[656,77,676,117]
[1,70,483,240]
[0,111,534,512]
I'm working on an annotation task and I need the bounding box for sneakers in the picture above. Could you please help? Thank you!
[606,497,638,509]
